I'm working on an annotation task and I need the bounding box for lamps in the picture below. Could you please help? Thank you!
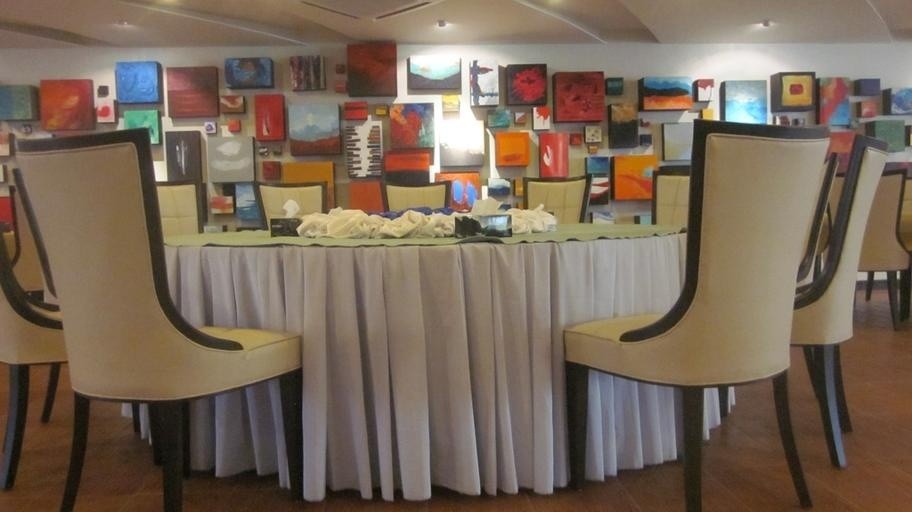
[439,20,446,27]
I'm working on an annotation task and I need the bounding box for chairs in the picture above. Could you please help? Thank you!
[522,174,591,223]
[0,154,61,311]
[0,232,68,493]
[647,165,692,234]
[250,179,326,228]
[381,180,451,212]
[5,126,307,511]
[561,116,832,512]
[825,158,910,329]
[788,133,891,471]
[157,180,206,237]
